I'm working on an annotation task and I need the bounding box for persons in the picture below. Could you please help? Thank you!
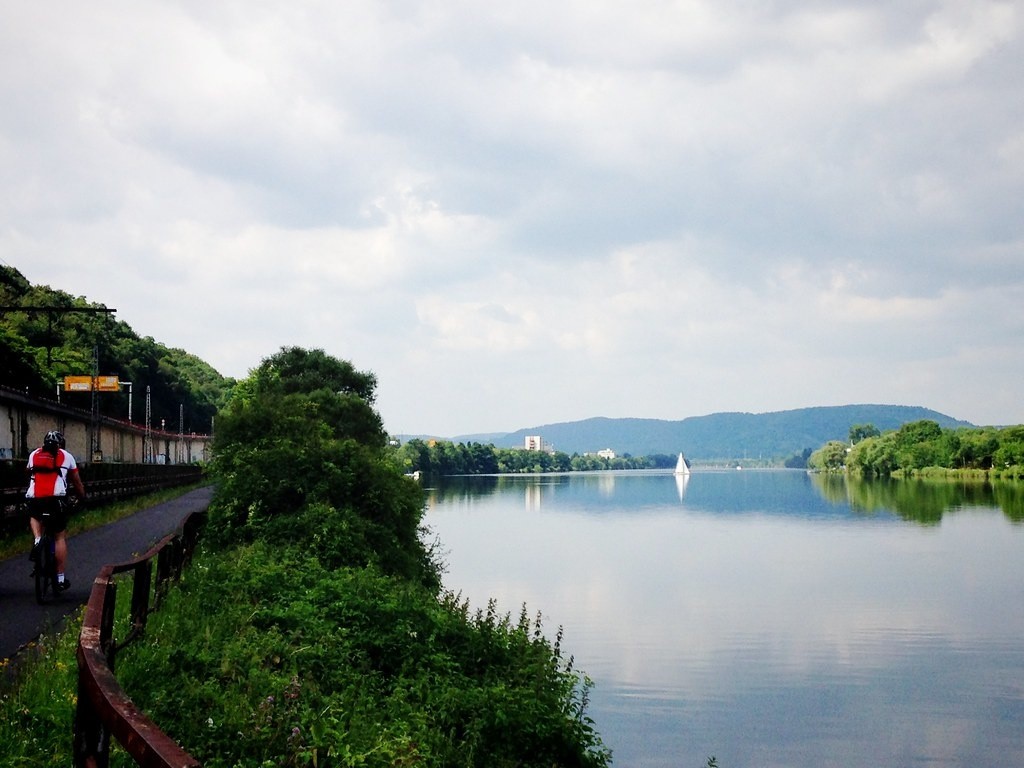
[25,430,92,591]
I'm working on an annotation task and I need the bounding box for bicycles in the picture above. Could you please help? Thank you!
[29,488,83,604]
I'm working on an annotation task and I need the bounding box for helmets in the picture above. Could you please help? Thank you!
[44,430,63,448]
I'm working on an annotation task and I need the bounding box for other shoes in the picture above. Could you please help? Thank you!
[56,576,71,592]
[28,541,43,563]
